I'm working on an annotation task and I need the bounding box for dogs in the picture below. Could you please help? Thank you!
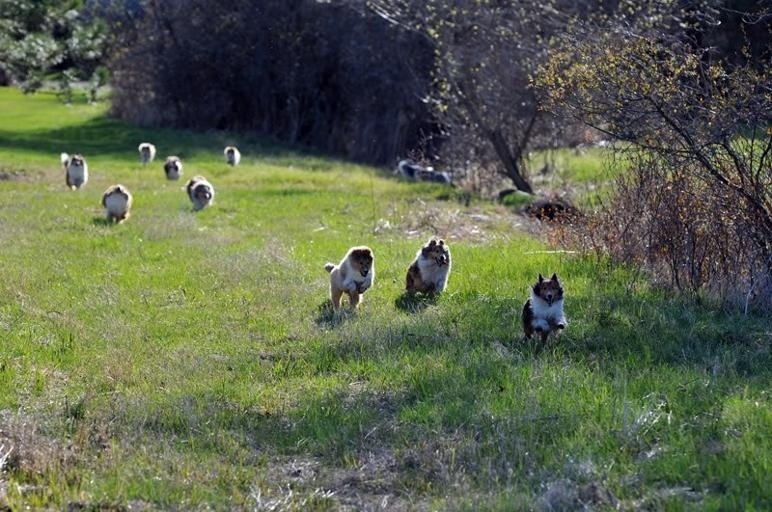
[164,155,182,180]
[139,142,156,164]
[325,246,374,313]
[522,273,568,360]
[406,238,452,303]
[224,146,241,167]
[61,153,89,192]
[183,176,214,212]
[102,185,132,225]
